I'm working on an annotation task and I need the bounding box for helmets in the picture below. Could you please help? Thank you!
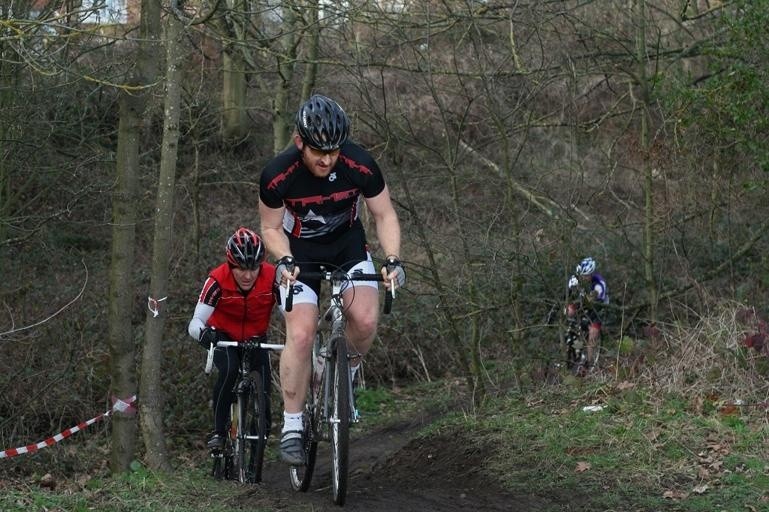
[575,258,598,277]
[294,94,350,153]
[224,227,266,270]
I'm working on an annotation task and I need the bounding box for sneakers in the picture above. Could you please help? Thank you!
[206,427,225,459]
[279,426,306,466]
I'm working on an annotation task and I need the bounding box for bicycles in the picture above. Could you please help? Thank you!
[275,254,400,508]
[204,326,285,486]
[563,301,605,380]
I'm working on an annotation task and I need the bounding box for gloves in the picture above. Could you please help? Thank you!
[197,326,220,350]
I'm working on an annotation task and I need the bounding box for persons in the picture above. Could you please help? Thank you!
[258,94,406,467]
[566,256,610,364]
[188,227,282,457]
